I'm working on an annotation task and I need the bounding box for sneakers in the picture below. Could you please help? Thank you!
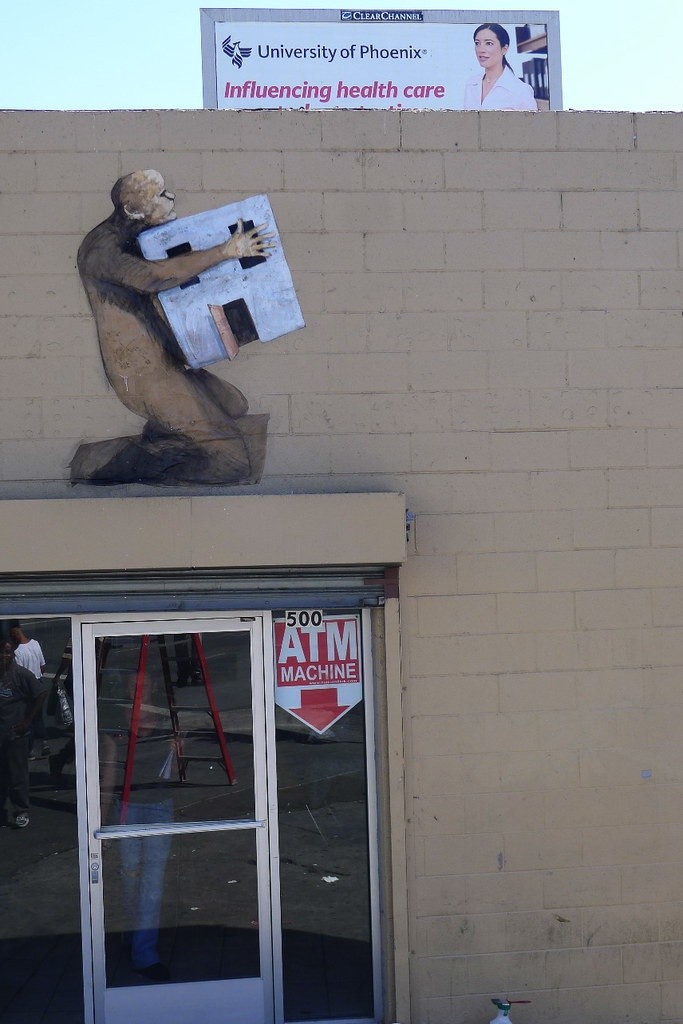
[42,740,51,756]
[28,749,35,760]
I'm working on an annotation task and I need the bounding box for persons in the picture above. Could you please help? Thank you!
[100,669,186,980]
[174,633,206,688]
[52,637,102,731]
[0,640,47,828]
[463,23,538,111]
[10,626,51,761]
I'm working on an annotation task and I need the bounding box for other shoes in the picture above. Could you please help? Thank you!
[132,963,176,984]
[177,676,188,688]
[49,754,65,779]
[192,671,200,676]
[16,812,29,828]
[121,917,130,944]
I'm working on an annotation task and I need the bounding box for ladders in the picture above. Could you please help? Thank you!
[97,633,239,826]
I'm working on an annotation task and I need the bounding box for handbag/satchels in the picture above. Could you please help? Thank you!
[47,682,56,715]
[57,682,73,727]
